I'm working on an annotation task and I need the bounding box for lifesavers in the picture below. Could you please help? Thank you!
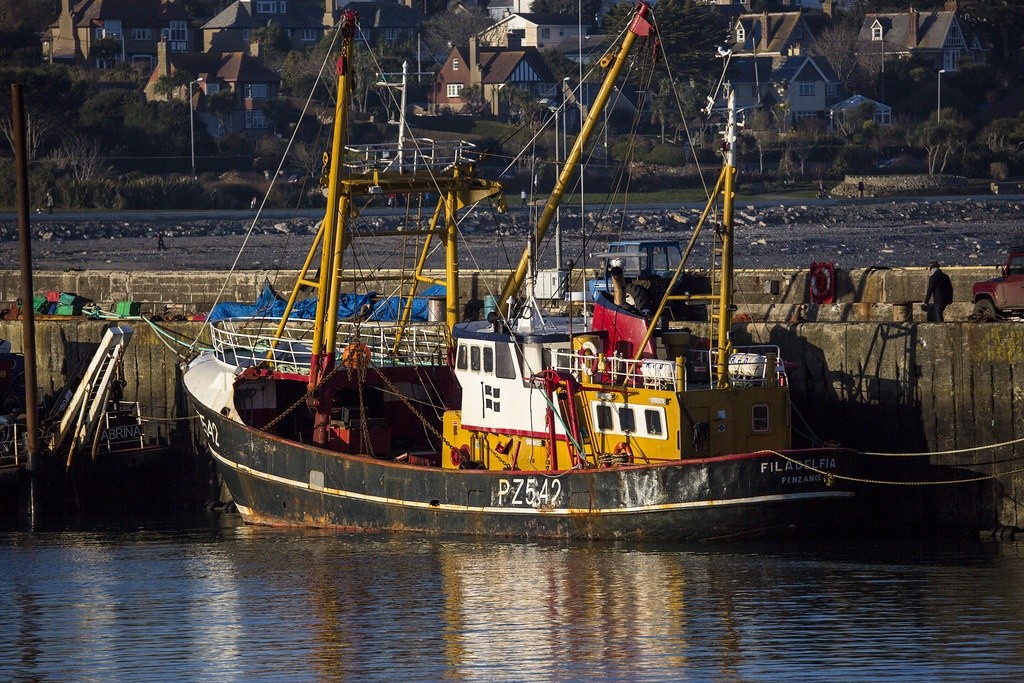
[577,341,598,376]
[811,267,833,298]
[613,441,634,468]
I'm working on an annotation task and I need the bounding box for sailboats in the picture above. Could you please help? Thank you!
[176,0,1013,554]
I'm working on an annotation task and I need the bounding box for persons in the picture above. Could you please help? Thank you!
[221,407,230,418]
[388,194,395,210]
[820,182,827,200]
[858,179,865,198]
[154,232,165,251]
[925,261,954,322]
[521,188,527,207]
[251,197,258,211]
[458,449,487,470]
[402,193,407,206]
[847,131,852,146]
[210,188,217,212]
[47,193,53,215]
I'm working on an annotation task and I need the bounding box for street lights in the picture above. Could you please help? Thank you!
[937,70,946,123]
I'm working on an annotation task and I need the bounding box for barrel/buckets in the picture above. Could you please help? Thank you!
[484,295,500,320]
[427,298,446,322]
